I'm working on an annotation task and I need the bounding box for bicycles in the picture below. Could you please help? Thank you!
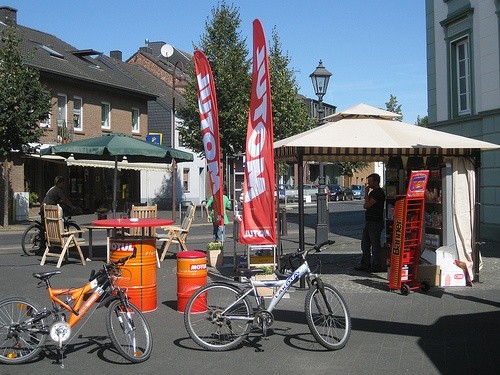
[20,206,83,256]
[184,241,352,352]
[0,247,154,363]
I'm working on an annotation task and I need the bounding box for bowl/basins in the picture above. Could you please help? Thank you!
[129,217,138,222]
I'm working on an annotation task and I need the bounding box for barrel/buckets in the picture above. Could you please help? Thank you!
[176,250,208,314]
[108,236,158,313]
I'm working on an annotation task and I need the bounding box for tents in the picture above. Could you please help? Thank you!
[262,103,500,285]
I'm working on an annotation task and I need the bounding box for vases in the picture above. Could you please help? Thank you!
[98,214,107,220]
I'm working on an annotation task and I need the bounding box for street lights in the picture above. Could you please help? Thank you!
[310,58,334,252]
[172,60,188,226]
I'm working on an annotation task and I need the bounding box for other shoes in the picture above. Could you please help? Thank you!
[353,267,365,271]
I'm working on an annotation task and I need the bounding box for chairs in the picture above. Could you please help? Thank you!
[40,203,86,269]
[132,203,161,269]
[159,204,196,262]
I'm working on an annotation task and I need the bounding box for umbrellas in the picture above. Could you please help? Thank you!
[38,132,193,243]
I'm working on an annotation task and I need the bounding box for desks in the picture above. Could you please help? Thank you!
[91,218,174,240]
[86,226,130,263]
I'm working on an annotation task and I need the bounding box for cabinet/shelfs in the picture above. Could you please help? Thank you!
[383,167,443,263]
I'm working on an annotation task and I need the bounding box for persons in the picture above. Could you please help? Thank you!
[204,189,232,249]
[353,173,385,271]
[40,175,77,245]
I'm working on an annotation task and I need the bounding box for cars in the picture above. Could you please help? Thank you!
[276,184,369,200]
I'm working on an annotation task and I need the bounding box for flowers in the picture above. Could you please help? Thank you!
[97,208,109,214]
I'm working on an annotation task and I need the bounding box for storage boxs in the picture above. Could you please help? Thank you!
[420,265,466,286]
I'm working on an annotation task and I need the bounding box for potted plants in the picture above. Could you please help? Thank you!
[61,127,73,144]
[255,265,275,297]
[207,241,224,266]
